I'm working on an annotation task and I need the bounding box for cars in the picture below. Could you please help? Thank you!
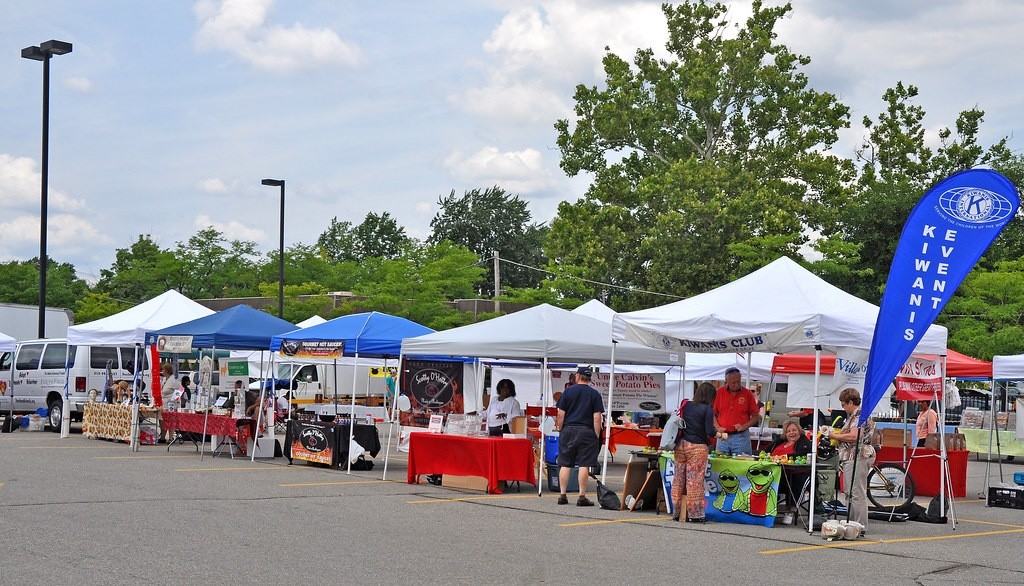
[894,381,1024,424]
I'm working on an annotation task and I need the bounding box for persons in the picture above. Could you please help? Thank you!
[916,400,937,447]
[180,375,191,409]
[766,421,819,525]
[714,366,760,455]
[828,388,877,535]
[750,384,764,427]
[384,368,398,422]
[158,365,179,444]
[105,379,132,405]
[277,390,294,417]
[670,382,717,522]
[557,366,605,506]
[788,407,827,430]
[224,380,270,457]
[466,378,520,489]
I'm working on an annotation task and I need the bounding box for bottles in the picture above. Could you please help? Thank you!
[398,393,411,412]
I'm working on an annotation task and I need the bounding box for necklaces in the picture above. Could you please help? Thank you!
[164,375,171,380]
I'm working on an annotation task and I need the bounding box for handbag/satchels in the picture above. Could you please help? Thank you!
[587,470,621,510]
[659,399,688,449]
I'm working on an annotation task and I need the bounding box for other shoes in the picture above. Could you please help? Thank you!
[167,437,175,445]
[673,516,679,520]
[576,498,595,506]
[556,498,569,503]
[782,513,795,525]
[689,517,708,523]
[157,438,166,443]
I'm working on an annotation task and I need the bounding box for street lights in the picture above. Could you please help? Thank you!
[261,179,285,319]
[20,40,74,339]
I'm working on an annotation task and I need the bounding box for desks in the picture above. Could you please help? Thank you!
[958,427,1024,462]
[406,430,536,495]
[875,422,958,446]
[159,410,257,460]
[81,400,158,450]
[627,449,835,541]
[285,418,380,470]
[305,404,390,423]
[874,443,969,499]
[599,425,669,463]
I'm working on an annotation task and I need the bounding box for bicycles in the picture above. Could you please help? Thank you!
[839,445,914,513]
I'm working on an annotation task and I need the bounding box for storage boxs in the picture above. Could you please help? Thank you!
[246,438,275,458]
[441,473,504,493]
[545,432,579,465]
[655,487,666,511]
[988,486,1024,510]
[18,417,46,431]
[545,463,579,491]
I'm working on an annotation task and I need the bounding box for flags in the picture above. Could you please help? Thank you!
[859,169,1020,428]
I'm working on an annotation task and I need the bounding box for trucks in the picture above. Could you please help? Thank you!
[249,362,398,422]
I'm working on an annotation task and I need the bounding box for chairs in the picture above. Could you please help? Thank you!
[524,402,559,444]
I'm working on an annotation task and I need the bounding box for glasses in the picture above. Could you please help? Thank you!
[750,390,755,393]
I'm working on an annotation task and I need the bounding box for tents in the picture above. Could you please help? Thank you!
[0,331,17,433]
[384,256,1024,520]
[251,311,438,474]
[135,302,303,461]
[60,288,217,453]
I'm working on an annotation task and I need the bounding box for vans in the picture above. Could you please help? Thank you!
[160,371,219,410]
[0,339,151,432]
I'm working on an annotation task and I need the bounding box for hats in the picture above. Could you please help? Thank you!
[574,367,592,377]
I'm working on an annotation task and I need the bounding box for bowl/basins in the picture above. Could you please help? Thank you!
[319,415,336,422]
